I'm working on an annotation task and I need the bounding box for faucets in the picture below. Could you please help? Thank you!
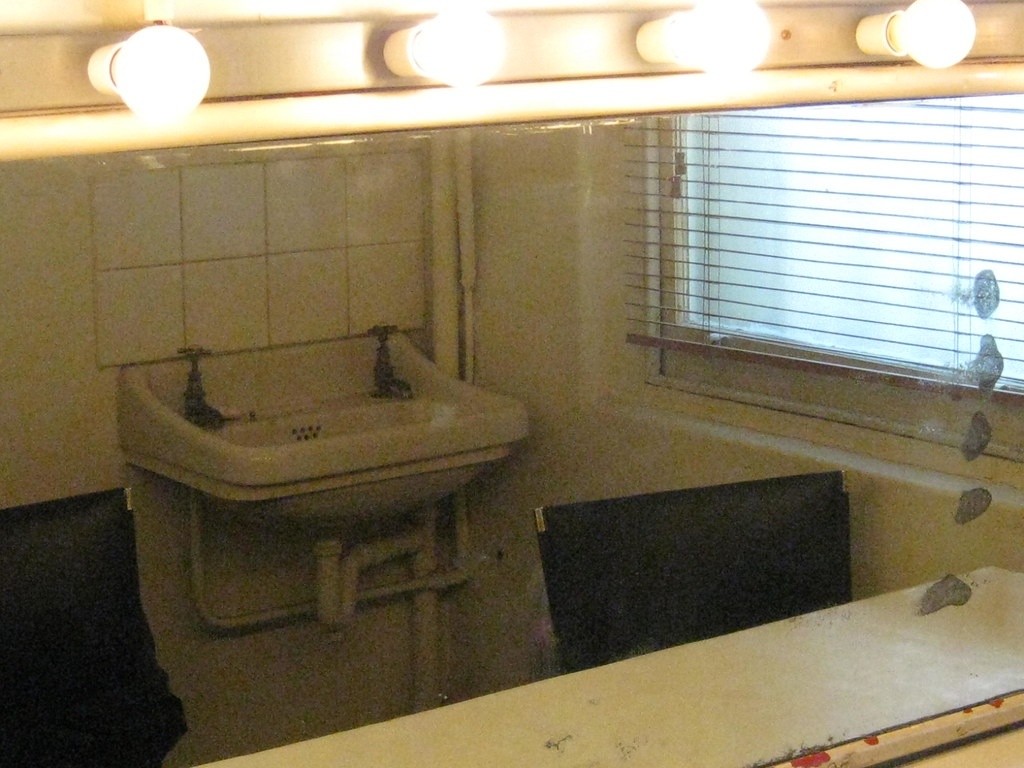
[366,324,415,399]
[176,343,227,429]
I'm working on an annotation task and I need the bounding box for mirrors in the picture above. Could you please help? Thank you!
[3,67,1024,768]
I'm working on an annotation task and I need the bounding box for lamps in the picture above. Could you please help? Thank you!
[855,0,978,67]
[382,9,510,85]
[87,22,213,123]
[635,2,777,75]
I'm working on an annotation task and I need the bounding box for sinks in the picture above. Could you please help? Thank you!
[118,331,538,527]
